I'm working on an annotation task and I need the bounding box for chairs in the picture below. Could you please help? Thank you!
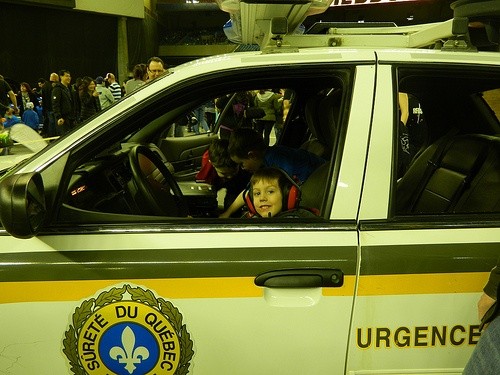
[299,94,341,213]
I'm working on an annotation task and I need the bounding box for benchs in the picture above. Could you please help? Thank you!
[410,133,500,215]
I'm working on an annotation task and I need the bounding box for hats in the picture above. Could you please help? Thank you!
[96,77,106,82]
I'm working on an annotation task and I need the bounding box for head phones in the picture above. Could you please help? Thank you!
[243,165,303,215]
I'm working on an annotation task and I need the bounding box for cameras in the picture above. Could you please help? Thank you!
[98,92,102,94]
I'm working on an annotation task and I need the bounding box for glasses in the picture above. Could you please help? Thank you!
[148,67,163,74]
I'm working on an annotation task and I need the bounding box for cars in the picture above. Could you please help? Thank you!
[0,47,500,375]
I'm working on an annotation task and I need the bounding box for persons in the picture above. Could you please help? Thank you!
[1,56,166,138]
[193,88,295,146]
[208,127,326,218]
[462,258,500,375]
